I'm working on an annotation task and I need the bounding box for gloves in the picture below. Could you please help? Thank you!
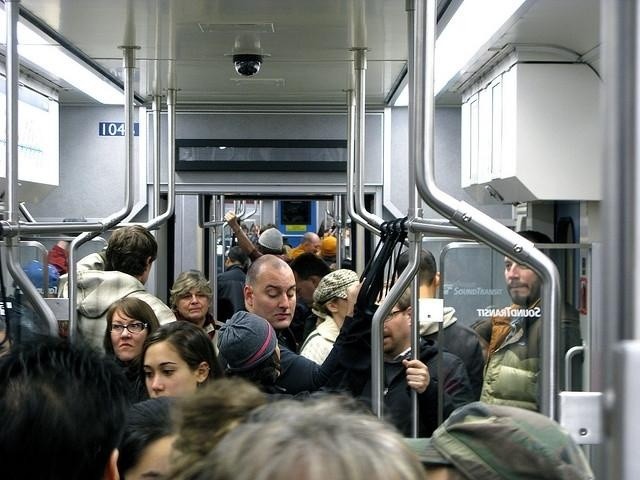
[61,218,86,240]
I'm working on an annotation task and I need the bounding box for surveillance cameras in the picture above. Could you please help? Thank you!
[224,31,271,77]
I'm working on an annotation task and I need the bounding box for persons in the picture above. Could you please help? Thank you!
[1,209,593,478]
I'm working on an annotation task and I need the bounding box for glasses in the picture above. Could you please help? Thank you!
[107,321,150,335]
[384,305,411,323]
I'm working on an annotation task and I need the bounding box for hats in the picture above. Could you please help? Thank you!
[320,236,338,257]
[16,258,59,296]
[405,399,598,480]
[258,227,284,251]
[311,269,361,311]
[215,310,279,374]
